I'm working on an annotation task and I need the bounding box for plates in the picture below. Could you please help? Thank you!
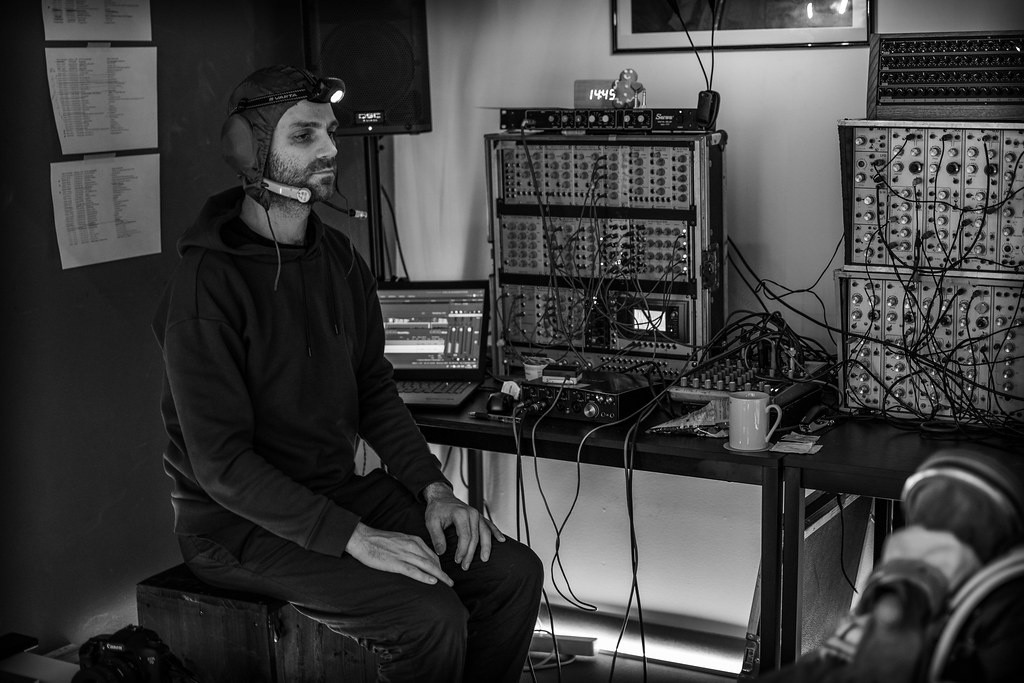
[723,442,775,453]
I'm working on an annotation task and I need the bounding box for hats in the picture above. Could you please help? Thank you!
[219,64,323,212]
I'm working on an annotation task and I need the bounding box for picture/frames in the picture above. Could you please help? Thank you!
[607,1,879,57]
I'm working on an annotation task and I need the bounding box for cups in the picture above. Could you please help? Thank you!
[728,391,782,452]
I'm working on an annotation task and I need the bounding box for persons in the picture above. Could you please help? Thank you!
[152,72,545,682]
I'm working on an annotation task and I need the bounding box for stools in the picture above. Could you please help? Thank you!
[135,563,302,681]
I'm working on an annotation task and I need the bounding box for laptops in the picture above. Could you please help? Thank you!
[375,280,490,412]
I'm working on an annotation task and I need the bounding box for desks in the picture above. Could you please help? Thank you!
[355,373,1023,682]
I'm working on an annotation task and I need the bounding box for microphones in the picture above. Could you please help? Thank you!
[317,196,367,218]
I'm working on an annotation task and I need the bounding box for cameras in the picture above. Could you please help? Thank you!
[71,623,182,683]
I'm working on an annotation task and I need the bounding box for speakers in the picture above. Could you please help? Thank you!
[302,0,432,137]
